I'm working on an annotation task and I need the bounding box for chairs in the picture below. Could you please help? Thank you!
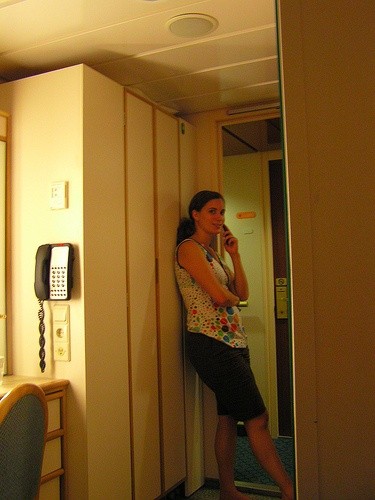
[0,384,49,500]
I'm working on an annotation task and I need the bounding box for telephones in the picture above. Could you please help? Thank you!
[34,243,73,300]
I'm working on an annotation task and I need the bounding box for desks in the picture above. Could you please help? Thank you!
[0,370,69,500]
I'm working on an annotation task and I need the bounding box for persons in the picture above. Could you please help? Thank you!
[173,190,296,500]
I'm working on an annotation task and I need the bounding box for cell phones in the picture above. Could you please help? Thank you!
[222,225,228,245]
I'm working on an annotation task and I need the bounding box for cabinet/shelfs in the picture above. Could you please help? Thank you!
[0,63,205,499]
